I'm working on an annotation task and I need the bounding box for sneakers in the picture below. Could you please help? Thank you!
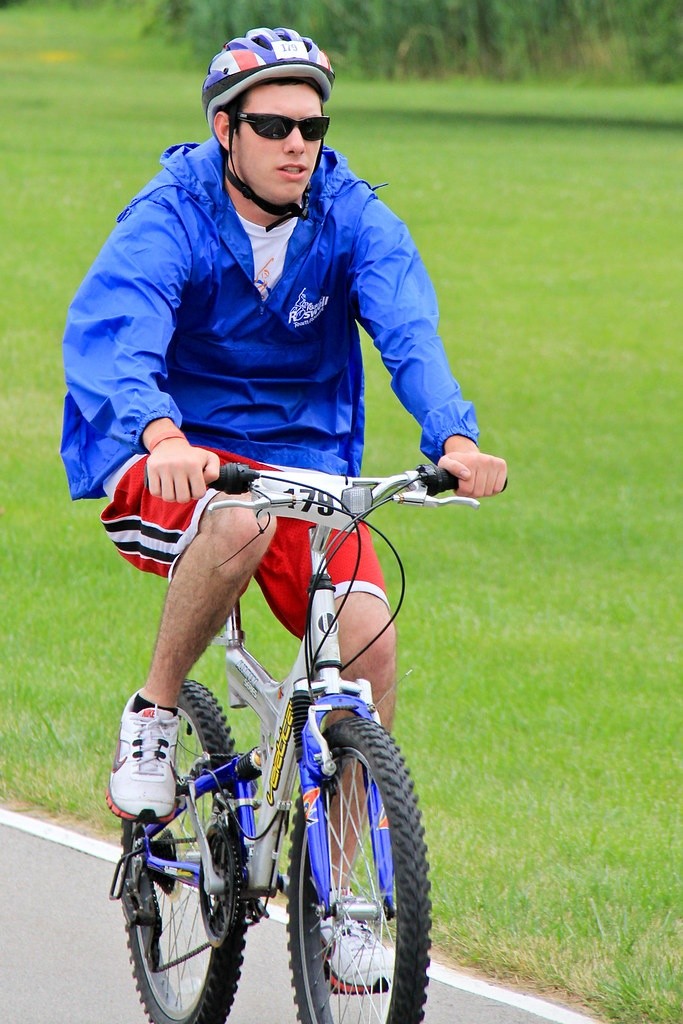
[106,686,180,825]
[322,888,391,996]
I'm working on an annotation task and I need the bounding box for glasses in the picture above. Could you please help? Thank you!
[235,111,331,141]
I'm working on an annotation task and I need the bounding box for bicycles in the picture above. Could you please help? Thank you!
[107,460,513,1022]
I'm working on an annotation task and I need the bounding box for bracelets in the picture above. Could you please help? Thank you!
[149,431,186,452]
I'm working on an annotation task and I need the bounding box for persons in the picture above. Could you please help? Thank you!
[58,27,507,996]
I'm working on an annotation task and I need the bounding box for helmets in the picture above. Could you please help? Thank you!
[202,27,336,151]
